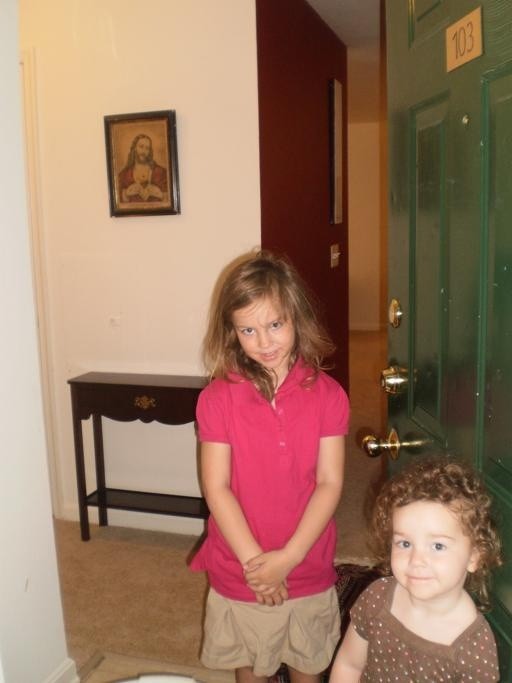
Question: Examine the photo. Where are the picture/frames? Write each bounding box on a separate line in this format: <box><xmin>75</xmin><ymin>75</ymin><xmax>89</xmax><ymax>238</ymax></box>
<box><xmin>103</xmin><ymin>109</ymin><xmax>182</xmax><ymax>219</ymax></box>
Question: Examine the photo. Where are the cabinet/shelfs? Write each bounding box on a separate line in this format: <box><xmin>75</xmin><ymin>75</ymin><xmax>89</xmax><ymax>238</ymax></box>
<box><xmin>67</xmin><ymin>370</ymin><xmax>215</xmax><ymax>543</ymax></box>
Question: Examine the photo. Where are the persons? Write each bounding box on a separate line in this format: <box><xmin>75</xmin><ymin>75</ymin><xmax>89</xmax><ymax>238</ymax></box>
<box><xmin>116</xmin><ymin>134</ymin><xmax>168</xmax><ymax>204</ymax></box>
<box><xmin>187</xmin><ymin>249</ymin><xmax>350</xmax><ymax>682</ymax></box>
<box><xmin>324</xmin><ymin>462</ymin><xmax>501</xmax><ymax>682</ymax></box>
